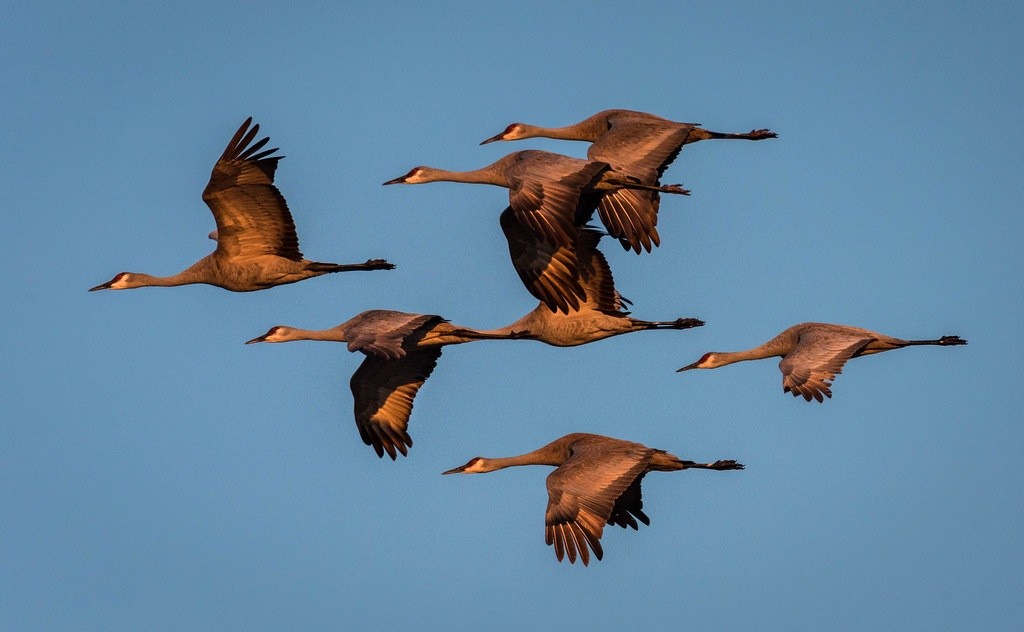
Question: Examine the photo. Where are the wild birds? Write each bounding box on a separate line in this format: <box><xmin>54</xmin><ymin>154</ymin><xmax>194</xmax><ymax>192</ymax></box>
<box><xmin>383</xmin><ymin>109</ymin><xmax>779</xmax><ymax>347</ymax></box>
<box><xmin>245</xmin><ymin>308</ymin><xmax>540</xmax><ymax>459</ymax></box>
<box><xmin>86</xmin><ymin>115</ymin><xmax>396</xmax><ymax>292</ymax></box>
<box><xmin>440</xmin><ymin>433</ymin><xmax>746</xmax><ymax>564</ymax></box>
<box><xmin>677</xmin><ymin>321</ymin><xmax>969</xmax><ymax>404</ymax></box>
